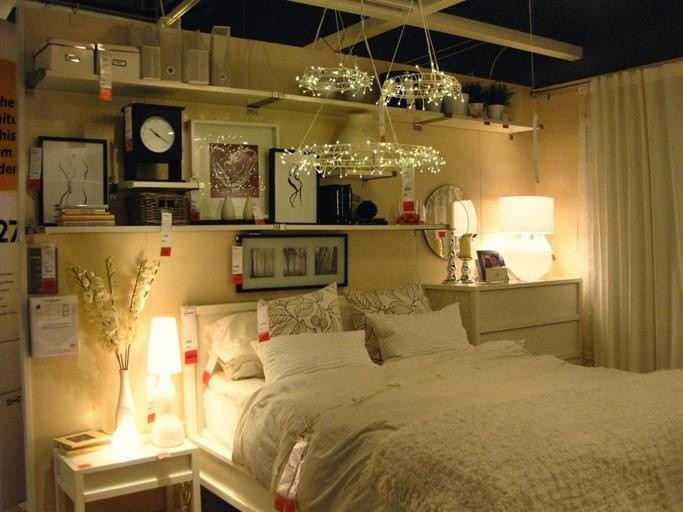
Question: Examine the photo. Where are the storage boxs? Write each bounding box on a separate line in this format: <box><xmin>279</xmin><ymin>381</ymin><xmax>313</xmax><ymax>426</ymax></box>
<box><xmin>95</xmin><ymin>43</ymin><xmax>140</xmax><ymax>80</ymax></box>
<box><xmin>33</xmin><ymin>38</ymin><xmax>95</xmax><ymax>76</ymax></box>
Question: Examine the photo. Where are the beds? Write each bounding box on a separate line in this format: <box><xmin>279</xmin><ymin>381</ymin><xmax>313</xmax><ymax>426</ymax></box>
<box><xmin>179</xmin><ymin>302</ymin><xmax>682</xmax><ymax>512</ymax></box>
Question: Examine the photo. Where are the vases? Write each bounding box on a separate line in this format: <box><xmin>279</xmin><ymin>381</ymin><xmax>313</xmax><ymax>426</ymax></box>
<box><xmin>242</xmin><ymin>196</ymin><xmax>254</xmax><ymax>220</ymax></box>
<box><xmin>422</xmin><ymin>95</ymin><xmax>444</xmax><ymax>113</ymax></box>
<box><xmin>442</xmin><ymin>92</ymin><xmax>470</xmax><ymax>117</ymax></box>
<box><xmin>221</xmin><ymin>195</ymin><xmax>235</xmax><ymax>220</ymax></box>
<box><xmin>114</xmin><ymin>368</ymin><xmax>137</xmax><ymax>435</ymax></box>
<box><xmin>338</xmin><ymin>86</ymin><xmax>366</xmax><ymax>104</ymax></box>
<box><xmin>374</xmin><ymin>70</ymin><xmax>423</xmax><ymax>111</ymax></box>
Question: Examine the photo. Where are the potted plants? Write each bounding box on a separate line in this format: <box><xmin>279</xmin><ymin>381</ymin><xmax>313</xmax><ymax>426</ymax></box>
<box><xmin>483</xmin><ymin>82</ymin><xmax>515</xmax><ymax>122</ymax></box>
<box><xmin>461</xmin><ymin>81</ymin><xmax>489</xmax><ymax>119</ymax></box>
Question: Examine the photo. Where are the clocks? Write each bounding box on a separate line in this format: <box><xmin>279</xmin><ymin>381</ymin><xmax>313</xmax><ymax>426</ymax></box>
<box><xmin>119</xmin><ymin>102</ymin><xmax>187</xmax><ymax>183</ymax></box>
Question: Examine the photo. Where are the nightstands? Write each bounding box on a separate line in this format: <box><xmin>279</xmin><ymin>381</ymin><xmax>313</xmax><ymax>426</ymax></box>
<box><xmin>421</xmin><ymin>278</ymin><xmax>583</xmax><ymax>366</ymax></box>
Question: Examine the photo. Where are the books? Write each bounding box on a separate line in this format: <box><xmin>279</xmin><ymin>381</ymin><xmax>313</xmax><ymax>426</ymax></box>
<box><xmin>53</xmin><ymin>202</ymin><xmax>115</xmax><ymax>225</ymax></box>
<box><xmin>53</xmin><ymin>430</ymin><xmax>111</xmax><ymax>458</ymax></box>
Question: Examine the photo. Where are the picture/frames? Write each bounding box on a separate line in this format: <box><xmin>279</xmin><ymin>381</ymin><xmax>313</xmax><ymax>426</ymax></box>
<box><xmin>268</xmin><ymin>149</ymin><xmax>319</xmax><ymax>225</ymax></box>
<box><xmin>38</xmin><ymin>136</ymin><xmax>108</xmax><ymax>227</ymax></box>
<box><xmin>475</xmin><ymin>249</ymin><xmax>510</xmax><ymax>282</ymax></box>
<box><xmin>235</xmin><ymin>233</ymin><xmax>348</xmax><ymax>294</ymax></box>
<box><xmin>189</xmin><ymin>118</ymin><xmax>280</xmax><ymax>220</ymax></box>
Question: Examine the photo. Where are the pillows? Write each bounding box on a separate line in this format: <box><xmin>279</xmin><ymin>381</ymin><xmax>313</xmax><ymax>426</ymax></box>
<box><xmin>203</xmin><ymin>311</ymin><xmax>264</xmax><ymax>381</ymax></box>
<box><xmin>256</xmin><ymin>281</ymin><xmax>343</xmax><ymax>340</ymax></box>
<box><xmin>342</xmin><ymin>278</ymin><xmax>428</xmax><ymax>363</ymax></box>
<box><xmin>248</xmin><ymin>329</ymin><xmax>372</xmax><ymax>384</ymax></box>
<box><xmin>365</xmin><ymin>302</ymin><xmax>469</xmax><ymax>366</ymax></box>
<box><xmin>337</xmin><ymin>295</ymin><xmax>353</xmax><ymax>331</ymax></box>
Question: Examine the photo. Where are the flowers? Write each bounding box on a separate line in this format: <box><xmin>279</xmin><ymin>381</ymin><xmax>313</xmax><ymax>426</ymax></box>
<box><xmin>66</xmin><ymin>257</ymin><xmax>160</xmax><ymax>370</ymax></box>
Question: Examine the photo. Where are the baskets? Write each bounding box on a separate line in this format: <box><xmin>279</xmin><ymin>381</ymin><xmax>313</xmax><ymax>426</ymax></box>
<box><xmin>125</xmin><ymin>193</ymin><xmax>189</xmax><ymax>224</ymax></box>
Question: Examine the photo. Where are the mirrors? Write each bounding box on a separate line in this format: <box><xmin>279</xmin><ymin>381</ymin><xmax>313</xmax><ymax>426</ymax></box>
<box><xmin>423</xmin><ymin>185</ymin><xmax>476</xmax><ymax>260</ymax></box>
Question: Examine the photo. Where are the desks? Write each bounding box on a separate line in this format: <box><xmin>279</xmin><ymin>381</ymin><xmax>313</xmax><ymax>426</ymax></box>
<box><xmin>51</xmin><ymin>431</ymin><xmax>202</xmax><ymax>512</ymax></box>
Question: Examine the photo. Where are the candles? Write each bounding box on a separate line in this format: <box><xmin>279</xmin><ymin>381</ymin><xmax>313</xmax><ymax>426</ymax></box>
<box><xmin>458</xmin><ymin>237</ymin><xmax>471</xmax><ymax>257</ymax></box>
<box><xmin>449</xmin><ymin>203</ymin><xmax>455</xmax><ymax>229</ymax></box>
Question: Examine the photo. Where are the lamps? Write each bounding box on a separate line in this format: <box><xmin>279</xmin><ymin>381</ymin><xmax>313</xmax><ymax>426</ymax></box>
<box><xmin>452</xmin><ymin>199</ymin><xmax>477</xmax><ymax>235</ymax></box>
<box><xmin>497</xmin><ymin>195</ymin><xmax>554</xmax><ymax>282</ymax></box>
<box><xmin>295</xmin><ymin>0</ymin><xmax>374</xmax><ymax>98</ymax></box>
<box><xmin>145</xmin><ymin>316</ymin><xmax>183</xmax><ymax>447</ymax></box>
<box><xmin>278</xmin><ymin>1</ymin><xmax>447</xmax><ymax>180</ymax></box>
<box><xmin>374</xmin><ymin>0</ymin><xmax>465</xmax><ymax>111</ymax></box>
